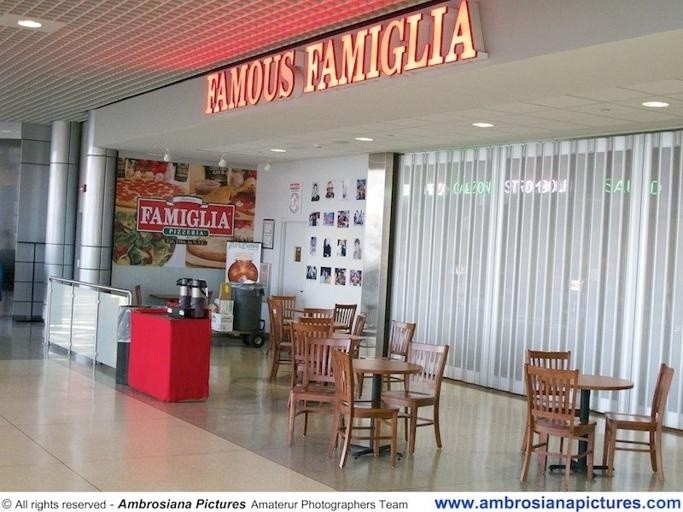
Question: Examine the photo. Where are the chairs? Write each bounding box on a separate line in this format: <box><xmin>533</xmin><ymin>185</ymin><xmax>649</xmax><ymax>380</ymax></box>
<box><xmin>265</xmin><ymin>296</ymin><xmax>448</xmax><ymax>470</ymax></box>
<box><xmin>520</xmin><ymin>352</ymin><xmax>674</xmax><ymax>482</ymax></box>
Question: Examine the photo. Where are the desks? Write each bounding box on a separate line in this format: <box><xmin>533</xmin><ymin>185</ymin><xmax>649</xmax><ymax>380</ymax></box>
<box><xmin>149</xmin><ymin>294</ymin><xmax>179</xmax><ymax>300</ymax></box>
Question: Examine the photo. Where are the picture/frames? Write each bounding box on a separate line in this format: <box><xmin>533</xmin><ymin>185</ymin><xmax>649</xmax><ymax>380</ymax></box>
<box><xmin>261</xmin><ymin>219</ymin><xmax>275</xmax><ymax>249</ymax></box>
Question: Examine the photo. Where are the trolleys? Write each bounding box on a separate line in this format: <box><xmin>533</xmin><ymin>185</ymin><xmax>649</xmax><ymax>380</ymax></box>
<box><xmin>210</xmin><ymin>319</ymin><xmax>266</xmax><ymax>348</ymax></box>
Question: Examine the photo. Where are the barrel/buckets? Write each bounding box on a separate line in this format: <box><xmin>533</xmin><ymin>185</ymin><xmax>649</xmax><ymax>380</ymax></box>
<box><xmin>231</xmin><ymin>284</ymin><xmax>265</xmax><ymax>332</ymax></box>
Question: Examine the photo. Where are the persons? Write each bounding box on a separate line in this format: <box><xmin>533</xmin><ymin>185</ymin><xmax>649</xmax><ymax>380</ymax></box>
<box><xmin>325</xmin><ymin>181</ymin><xmax>335</xmax><ymax>198</ymax></box>
<box><xmin>351</xmin><ymin>239</ymin><xmax>362</xmax><ymax>261</ymax></box>
<box><xmin>312</xmin><ymin>183</ymin><xmax>320</xmax><ymax>201</ymax></box>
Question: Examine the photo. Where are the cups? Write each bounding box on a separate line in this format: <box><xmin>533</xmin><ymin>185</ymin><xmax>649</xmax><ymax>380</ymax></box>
<box><xmin>192</xmin><ymin>180</ymin><xmax>221</xmax><ymax>195</ymax></box>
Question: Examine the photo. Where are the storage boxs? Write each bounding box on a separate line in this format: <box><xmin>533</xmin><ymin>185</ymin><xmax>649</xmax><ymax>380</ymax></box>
<box><xmin>211</xmin><ymin>298</ymin><xmax>234</xmax><ymax>332</ymax></box>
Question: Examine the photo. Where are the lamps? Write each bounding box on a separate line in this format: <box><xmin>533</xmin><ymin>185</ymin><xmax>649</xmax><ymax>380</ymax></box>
<box><xmin>164</xmin><ymin>151</ymin><xmax>273</xmax><ymax>172</ymax></box>
